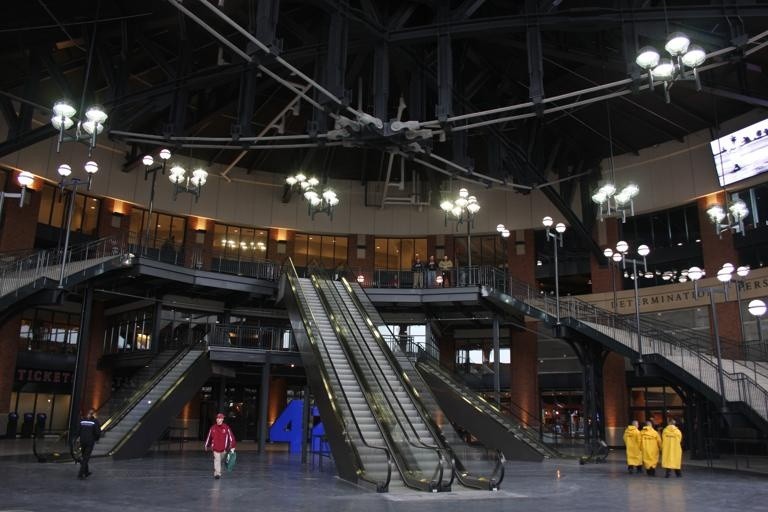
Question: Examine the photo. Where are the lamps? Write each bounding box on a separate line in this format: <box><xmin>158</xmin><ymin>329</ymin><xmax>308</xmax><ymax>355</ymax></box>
<box><xmin>706</xmin><ymin>199</ymin><xmax>750</xmax><ymax>233</ymax></box>
<box><xmin>542</xmin><ymin>216</ymin><xmax>554</xmax><ymax>228</ymax></box>
<box><xmin>592</xmin><ymin>183</ymin><xmax>641</xmax><ymax>206</ymax></box>
<box><xmin>285</xmin><ymin>173</ymin><xmax>340</xmax><ymax>207</ymax></box>
<box><xmin>440</xmin><ymin>185</ymin><xmax>483</xmax><ymax>222</ymax></box>
<box><xmin>17</xmin><ymin>103</ymin><xmax>209</xmax><ymax>188</ymax></box>
<box><xmin>635</xmin><ymin>32</ymin><xmax>707</xmax><ymax>82</ymax></box>
<box><xmin>555</xmin><ymin>222</ymin><xmax>566</xmax><ymax>233</ymax></box>
<box><xmin>496</xmin><ymin>223</ymin><xmax>511</xmax><ymax>238</ymax></box>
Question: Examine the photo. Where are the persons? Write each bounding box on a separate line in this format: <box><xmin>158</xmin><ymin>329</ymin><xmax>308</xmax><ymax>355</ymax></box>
<box><xmin>425</xmin><ymin>254</ymin><xmax>439</xmax><ymax>289</ymax></box>
<box><xmin>640</xmin><ymin>420</ymin><xmax>663</xmax><ymax>476</ymax></box>
<box><xmin>412</xmin><ymin>255</ymin><xmax>426</xmax><ymax>289</ymax></box>
<box><xmin>622</xmin><ymin>419</ymin><xmax>644</xmax><ymax>475</ymax></box>
<box><xmin>438</xmin><ymin>254</ymin><xmax>453</xmax><ymax>288</ymax></box>
<box><xmin>73</xmin><ymin>407</ymin><xmax>102</xmax><ymax>480</ymax></box>
<box><xmin>205</xmin><ymin>413</ymin><xmax>237</xmax><ymax>480</ymax></box>
<box><xmin>660</xmin><ymin>417</ymin><xmax>683</xmax><ymax>479</ymax></box>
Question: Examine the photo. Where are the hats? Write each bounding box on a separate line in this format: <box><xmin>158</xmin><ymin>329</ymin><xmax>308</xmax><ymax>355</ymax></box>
<box><xmin>216</xmin><ymin>414</ymin><xmax>224</xmax><ymax>418</ymax></box>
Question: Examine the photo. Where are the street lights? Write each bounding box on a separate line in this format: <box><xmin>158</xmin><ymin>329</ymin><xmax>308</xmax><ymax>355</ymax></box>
<box><xmin>459</xmin><ymin>187</ymin><xmax>478</xmax><ymax>285</ymax></box>
<box><xmin>543</xmin><ymin>215</ymin><xmax>566</xmax><ymax>326</ymax></box>
<box><xmin>143</xmin><ymin>148</ymin><xmax>172</xmax><ymax>256</ymax></box>
<box><xmin>496</xmin><ymin>223</ymin><xmax>511</xmax><ymax>294</ymax></box>
<box><xmin>686</xmin><ymin>266</ymin><xmax>732</xmax><ymax>414</ymax></box>
<box><xmin>722</xmin><ymin>262</ymin><xmax>750</xmax><ymax>361</ymax></box>
<box><xmin>56</xmin><ymin>160</ymin><xmax>99</xmax><ymax>289</ymax></box>
<box><xmin>603</xmin><ymin>246</ymin><xmax>622</xmax><ymax>315</ymax></box>
<box><xmin>616</xmin><ymin>240</ymin><xmax>651</xmax><ymax>360</ymax></box>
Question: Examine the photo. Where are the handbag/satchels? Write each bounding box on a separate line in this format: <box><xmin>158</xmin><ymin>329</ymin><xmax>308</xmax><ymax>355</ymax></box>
<box><xmin>226</xmin><ymin>451</ymin><xmax>236</xmax><ymax>472</ymax></box>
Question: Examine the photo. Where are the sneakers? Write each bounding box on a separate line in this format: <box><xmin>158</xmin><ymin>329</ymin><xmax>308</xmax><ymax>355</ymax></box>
<box><xmin>215</xmin><ymin>475</ymin><xmax>220</xmax><ymax>479</ymax></box>
<box><xmin>628</xmin><ymin>471</ymin><xmax>681</xmax><ymax>478</ymax></box>
